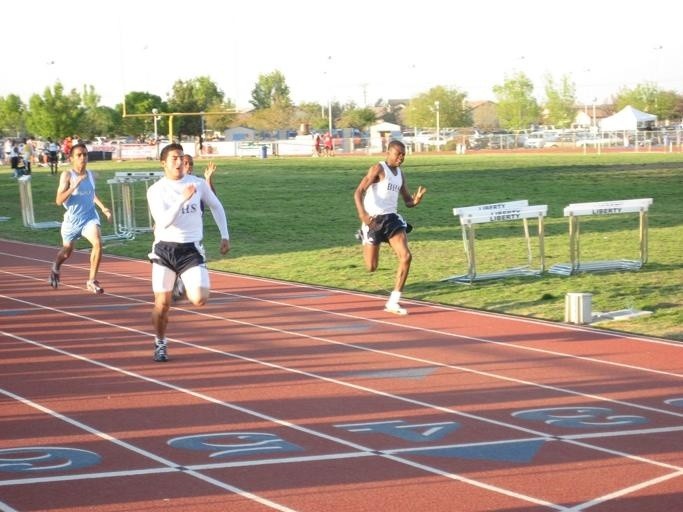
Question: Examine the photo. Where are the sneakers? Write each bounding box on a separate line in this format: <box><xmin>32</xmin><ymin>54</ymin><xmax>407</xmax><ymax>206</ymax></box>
<box><xmin>383</xmin><ymin>301</ymin><xmax>409</xmax><ymax>316</ymax></box>
<box><xmin>87</xmin><ymin>280</ymin><xmax>104</xmax><ymax>296</ymax></box>
<box><xmin>355</xmin><ymin>221</ymin><xmax>370</xmax><ymax>241</ymax></box>
<box><xmin>49</xmin><ymin>264</ymin><xmax>61</xmax><ymax>289</ymax></box>
<box><xmin>153</xmin><ymin>337</ymin><xmax>168</xmax><ymax>362</ymax></box>
<box><xmin>171</xmin><ymin>276</ymin><xmax>186</xmax><ymax>303</ymax></box>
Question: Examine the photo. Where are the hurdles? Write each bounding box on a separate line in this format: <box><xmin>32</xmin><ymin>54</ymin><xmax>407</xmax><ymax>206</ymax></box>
<box><xmin>551</xmin><ymin>198</ymin><xmax>655</xmax><ymax>276</ymax></box>
<box><xmin>452</xmin><ymin>200</ymin><xmax>549</xmax><ymax>284</ymax></box>
<box><xmin>107</xmin><ymin>169</ymin><xmax>169</xmax><ymax>238</ymax></box>
<box><xmin>17</xmin><ymin>174</ymin><xmax>61</xmax><ymax>230</ymax></box>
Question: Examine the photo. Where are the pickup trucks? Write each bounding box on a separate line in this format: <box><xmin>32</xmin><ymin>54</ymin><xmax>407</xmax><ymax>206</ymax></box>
<box><xmin>103</xmin><ymin>136</ymin><xmax>135</xmax><ymax>145</ymax></box>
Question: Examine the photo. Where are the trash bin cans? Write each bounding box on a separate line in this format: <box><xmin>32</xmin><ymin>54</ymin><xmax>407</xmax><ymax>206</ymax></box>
<box><xmin>260</xmin><ymin>147</ymin><xmax>266</xmax><ymax>158</ymax></box>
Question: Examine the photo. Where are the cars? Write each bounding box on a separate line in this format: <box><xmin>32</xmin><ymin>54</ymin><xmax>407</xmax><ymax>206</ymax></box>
<box><xmin>403</xmin><ymin>124</ymin><xmax>622</xmax><ymax>149</ymax></box>
<box><xmin>333</xmin><ymin>129</ymin><xmax>362</xmax><ymax>148</ymax></box>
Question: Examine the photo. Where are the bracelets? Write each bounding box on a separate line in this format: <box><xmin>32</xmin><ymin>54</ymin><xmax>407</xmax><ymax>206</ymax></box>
<box><xmin>101</xmin><ymin>207</ymin><xmax>110</xmax><ymax>216</ymax></box>
<box><xmin>70</xmin><ymin>182</ymin><xmax>78</xmax><ymax>189</ymax></box>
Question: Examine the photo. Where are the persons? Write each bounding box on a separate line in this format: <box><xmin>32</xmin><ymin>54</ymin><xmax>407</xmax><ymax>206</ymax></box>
<box><xmin>138</xmin><ymin>132</ymin><xmax>231</xmax><ymax>362</ymax></box>
<box><xmin>0</xmin><ymin>134</ymin><xmax>84</xmax><ymax>178</ymax></box>
<box><xmin>313</xmin><ymin>131</ymin><xmax>335</xmax><ymax>156</ymax></box>
<box><xmin>47</xmin><ymin>144</ymin><xmax>113</xmax><ymax>295</ymax></box>
<box><xmin>353</xmin><ymin>139</ymin><xmax>428</xmax><ymax>316</ymax></box>
